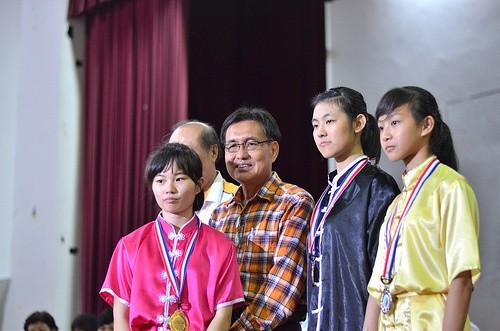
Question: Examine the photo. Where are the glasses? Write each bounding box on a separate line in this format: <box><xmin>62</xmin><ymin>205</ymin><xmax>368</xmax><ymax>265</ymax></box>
<box><xmin>225</xmin><ymin>139</ymin><xmax>274</xmax><ymax>153</ymax></box>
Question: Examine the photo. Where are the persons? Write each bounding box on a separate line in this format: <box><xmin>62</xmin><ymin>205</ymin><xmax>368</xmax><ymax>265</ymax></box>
<box><xmin>71</xmin><ymin>307</ymin><xmax>114</xmax><ymax>331</ymax></box>
<box><xmin>99</xmin><ymin>142</ymin><xmax>245</xmax><ymax>331</ymax></box>
<box><xmin>306</xmin><ymin>86</ymin><xmax>402</xmax><ymax>331</ymax></box>
<box><xmin>361</xmin><ymin>86</ymin><xmax>481</xmax><ymax>331</ymax></box>
<box><xmin>168</xmin><ymin>120</ymin><xmax>239</xmax><ymax>226</ymax></box>
<box><xmin>208</xmin><ymin>108</ymin><xmax>316</xmax><ymax>331</ymax></box>
<box><xmin>24</xmin><ymin>311</ymin><xmax>59</xmax><ymax>331</ymax></box>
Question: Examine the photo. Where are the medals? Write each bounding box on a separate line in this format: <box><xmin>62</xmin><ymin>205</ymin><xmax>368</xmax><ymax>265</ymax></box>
<box><xmin>378</xmin><ymin>288</ymin><xmax>393</xmax><ymax>313</ymax></box>
<box><xmin>308</xmin><ymin>260</ymin><xmax>318</xmax><ymax>283</ymax></box>
<box><xmin>169</xmin><ymin>308</ymin><xmax>189</xmax><ymax>331</ymax></box>
